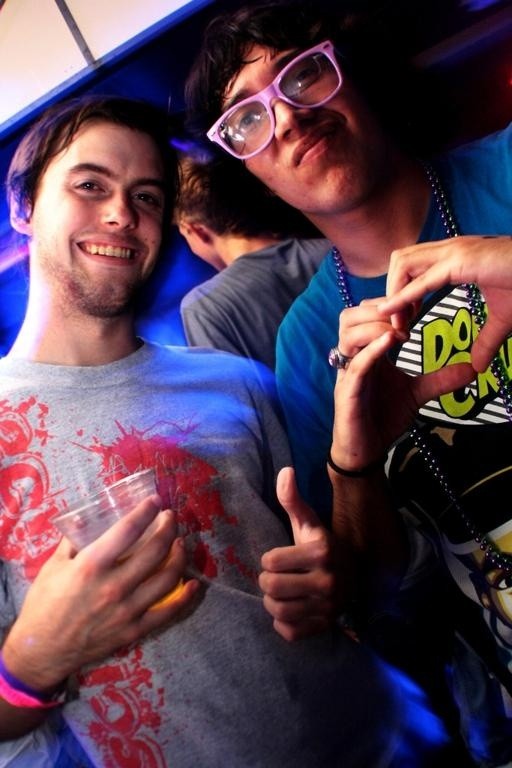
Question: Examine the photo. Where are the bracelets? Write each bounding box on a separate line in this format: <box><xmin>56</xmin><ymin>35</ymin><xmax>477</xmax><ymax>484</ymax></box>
<box><xmin>0</xmin><ymin>647</ymin><xmax>68</xmax><ymax>713</ymax></box>
<box><xmin>326</xmin><ymin>447</ymin><xmax>371</xmax><ymax>483</ymax></box>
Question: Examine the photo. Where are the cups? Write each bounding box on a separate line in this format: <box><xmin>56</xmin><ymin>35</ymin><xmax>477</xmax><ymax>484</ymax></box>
<box><xmin>49</xmin><ymin>468</ymin><xmax>185</xmax><ymax>614</ymax></box>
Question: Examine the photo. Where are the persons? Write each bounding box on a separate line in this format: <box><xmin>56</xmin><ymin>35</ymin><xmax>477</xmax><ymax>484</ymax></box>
<box><xmin>169</xmin><ymin>3</ymin><xmax>511</xmax><ymax>710</ymax></box>
<box><xmin>167</xmin><ymin>147</ymin><xmax>511</xmax><ymax>764</ymax></box>
<box><xmin>0</xmin><ymin>95</ymin><xmax>411</xmax><ymax>765</ymax></box>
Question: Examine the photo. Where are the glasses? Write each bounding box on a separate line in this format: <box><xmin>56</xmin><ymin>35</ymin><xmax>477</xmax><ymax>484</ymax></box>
<box><xmin>204</xmin><ymin>40</ymin><xmax>345</xmax><ymax>160</ymax></box>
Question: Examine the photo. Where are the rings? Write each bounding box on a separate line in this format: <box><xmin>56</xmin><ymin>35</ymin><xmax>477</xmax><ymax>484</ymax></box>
<box><xmin>324</xmin><ymin>349</ymin><xmax>353</xmax><ymax>370</ymax></box>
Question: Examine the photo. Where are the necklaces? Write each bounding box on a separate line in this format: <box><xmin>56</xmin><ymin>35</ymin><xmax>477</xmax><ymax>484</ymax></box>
<box><xmin>328</xmin><ymin>163</ymin><xmax>510</xmax><ymax>577</ymax></box>
